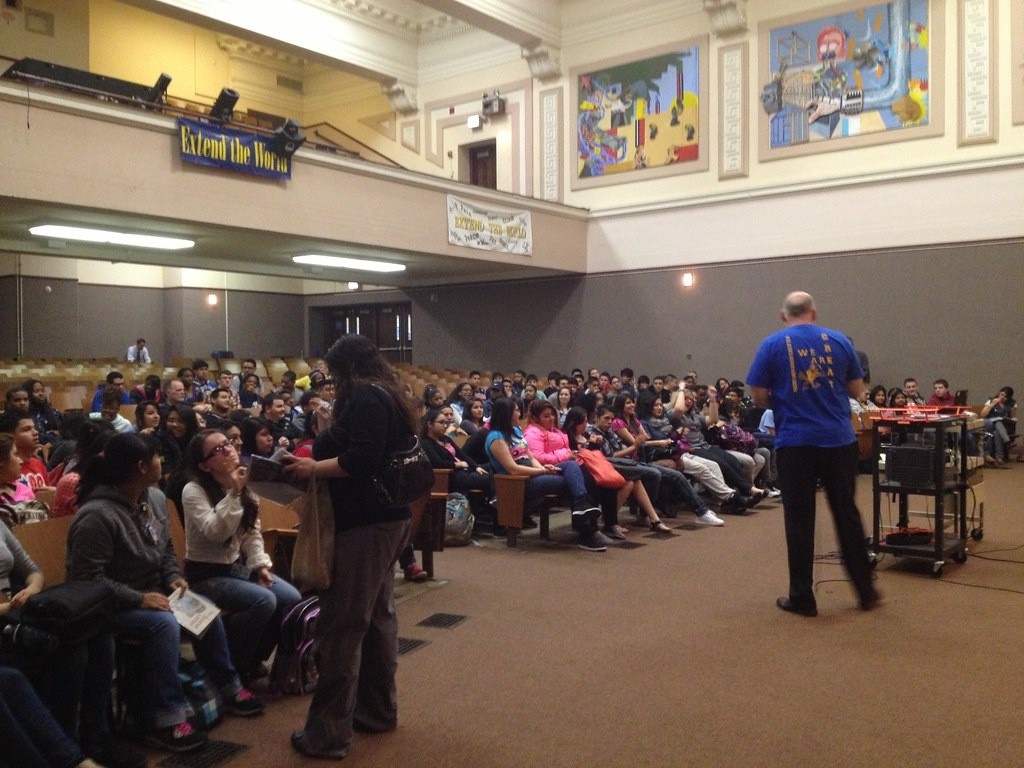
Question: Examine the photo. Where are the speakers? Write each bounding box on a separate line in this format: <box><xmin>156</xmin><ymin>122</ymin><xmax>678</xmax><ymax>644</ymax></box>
<box><xmin>885</xmin><ymin>442</ymin><xmax>937</xmax><ymax>487</ymax></box>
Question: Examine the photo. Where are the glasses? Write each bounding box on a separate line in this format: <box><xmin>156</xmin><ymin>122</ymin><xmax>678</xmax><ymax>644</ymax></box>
<box><xmin>431</xmin><ymin>419</ymin><xmax>449</xmax><ymax>426</ymax></box>
<box><xmin>201</xmin><ymin>438</ymin><xmax>235</xmax><ymax>464</ymax></box>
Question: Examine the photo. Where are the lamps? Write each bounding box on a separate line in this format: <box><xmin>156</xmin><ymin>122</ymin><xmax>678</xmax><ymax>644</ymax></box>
<box><xmin>480</xmin><ymin>89</ymin><xmax>505</xmax><ymax>115</ymax></box>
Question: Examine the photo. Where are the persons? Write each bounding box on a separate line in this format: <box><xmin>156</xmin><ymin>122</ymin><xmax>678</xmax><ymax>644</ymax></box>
<box><xmin>280</xmin><ymin>334</ymin><xmax>413</xmax><ymax>757</ymax></box>
<box><xmin>842</xmin><ymin>336</ymin><xmax>1017</xmax><ymax>468</ymax></box>
<box><xmin>128</xmin><ymin>338</ymin><xmax>152</xmax><ymax>364</ymax></box>
<box><xmin>746</xmin><ymin>292</ymin><xmax>887</xmax><ymax>617</ymax></box>
<box><xmin>0</xmin><ymin>359</ymin><xmax>776</xmax><ymax>768</ymax></box>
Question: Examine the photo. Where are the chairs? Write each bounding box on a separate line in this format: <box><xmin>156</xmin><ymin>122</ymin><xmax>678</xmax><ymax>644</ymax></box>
<box><xmin>0</xmin><ymin>347</ymin><xmax>534</xmax><ymax>589</ymax></box>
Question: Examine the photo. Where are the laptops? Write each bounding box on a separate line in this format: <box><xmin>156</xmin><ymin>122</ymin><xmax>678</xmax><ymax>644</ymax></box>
<box><xmin>954</xmin><ymin>389</ymin><xmax>968</xmax><ymax>406</ymax></box>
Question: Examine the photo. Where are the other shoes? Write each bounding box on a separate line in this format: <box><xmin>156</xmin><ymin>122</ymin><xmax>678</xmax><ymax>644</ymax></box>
<box><xmin>594</xmin><ymin>530</ymin><xmax>614</xmax><ymax>544</ymax></box>
<box><xmin>90</xmin><ymin>743</ymin><xmax>148</xmax><ymax>768</ymax></box>
<box><xmin>403</xmin><ymin>564</ymin><xmax>428</xmax><ymax>581</ymax></box>
<box><xmin>492</xmin><ymin>525</ymin><xmax>523</xmax><ymax>539</ymax></box>
<box><xmin>767</xmin><ymin>486</ymin><xmax>781</xmax><ymax>497</ymax></box>
<box><xmin>222</xmin><ymin>689</ymin><xmax>265</xmax><ymax>716</ymax></box>
<box><xmin>577</xmin><ymin>536</ymin><xmax>606</xmax><ymax>551</ymax></box>
<box><xmin>572</xmin><ymin>494</ymin><xmax>603</xmax><ymax>523</ymax></box>
<box><xmin>489</xmin><ymin>496</ymin><xmax>498</xmax><ymax>509</ymax></box>
<box><xmin>637</xmin><ymin>514</ymin><xmax>651</xmax><ymax>526</ymax></box>
<box><xmin>776</xmin><ymin>597</ymin><xmax>818</xmax><ymax>617</ymax></box>
<box><xmin>523</xmin><ymin>513</ymin><xmax>537</xmax><ymax>527</ymax></box>
<box><xmin>695</xmin><ymin>509</ymin><xmax>725</xmax><ymax>526</ymax></box>
<box><xmin>604</xmin><ymin>525</ymin><xmax>626</xmax><ymax>539</ymax></box>
<box><xmin>145</xmin><ymin>722</ymin><xmax>204</xmax><ymax>752</ymax></box>
<box><xmin>720</xmin><ymin>490</ymin><xmax>762</xmax><ymax>514</ymax></box>
<box><xmin>237</xmin><ymin>657</ymin><xmax>268</xmax><ymax>684</ymax></box>
<box><xmin>750</xmin><ymin>485</ymin><xmax>768</xmax><ymax>499</ymax></box>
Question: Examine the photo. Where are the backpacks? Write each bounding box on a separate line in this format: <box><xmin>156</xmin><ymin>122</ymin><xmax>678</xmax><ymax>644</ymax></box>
<box><xmin>441</xmin><ymin>491</ymin><xmax>475</xmax><ymax>547</ymax></box>
<box><xmin>277</xmin><ymin>592</ymin><xmax>322</xmax><ymax>695</ymax></box>
<box><xmin>721</xmin><ymin>423</ymin><xmax>759</xmax><ymax>453</ymax></box>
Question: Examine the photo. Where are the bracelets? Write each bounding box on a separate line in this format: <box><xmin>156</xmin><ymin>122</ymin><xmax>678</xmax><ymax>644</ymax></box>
<box><xmin>678</xmin><ymin>389</ymin><xmax>685</xmax><ymax>392</ymax></box>
<box><xmin>460</xmin><ymin>461</ymin><xmax>464</xmax><ymax>468</ymax></box>
<box><xmin>541</xmin><ymin>466</ymin><xmax>545</xmax><ymax>475</ymax></box>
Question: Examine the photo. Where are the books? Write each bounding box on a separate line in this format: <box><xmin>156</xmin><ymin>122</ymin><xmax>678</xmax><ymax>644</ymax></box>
<box><xmin>166</xmin><ymin>586</ymin><xmax>222</xmax><ymax>642</ymax></box>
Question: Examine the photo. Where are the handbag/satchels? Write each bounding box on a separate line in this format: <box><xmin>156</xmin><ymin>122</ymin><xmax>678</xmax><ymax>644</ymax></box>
<box><xmin>576</xmin><ymin>446</ymin><xmax>626</xmax><ymax>489</ymax></box>
<box><xmin>18</xmin><ymin>577</ymin><xmax>121</xmax><ymax>651</ymax></box>
<box><xmin>645</xmin><ymin>429</ymin><xmax>681</xmax><ymax>463</ymax></box>
<box><xmin>289</xmin><ymin>462</ymin><xmax>337</xmax><ymax>593</ymax></box>
<box><xmin>122</xmin><ymin>651</ymin><xmax>226</xmax><ymax>732</ymax></box>
<box><xmin>353</xmin><ymin>382</ymin><xmax>437</xmax><ymax>510</ymax></box>
<box><xmin>661</xmin><ymin>429</ymin><xmax>690</xmax><ymax>461</ymax></box>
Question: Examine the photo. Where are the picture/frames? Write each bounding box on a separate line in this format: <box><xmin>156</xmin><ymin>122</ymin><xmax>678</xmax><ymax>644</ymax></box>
<box><xmin>757</xmin><ymin>0</ymin><xmax>946</xmax><ymax>163</ymax></box>
<box><xmin>570</xmin><ymin>33</ymin><xmax>710</xmax><ymax>191</ymax></box>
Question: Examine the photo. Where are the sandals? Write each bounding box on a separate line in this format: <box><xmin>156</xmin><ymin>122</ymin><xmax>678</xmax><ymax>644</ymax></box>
<box><xmin>649</xmin><ymin>521</ymin><xmax>672</xmax><ymax>532</ymax></box>
<box><xmin>618</xmin><ymin>523</ymin><xmax>630</xmax><ymax>532</ymax></box>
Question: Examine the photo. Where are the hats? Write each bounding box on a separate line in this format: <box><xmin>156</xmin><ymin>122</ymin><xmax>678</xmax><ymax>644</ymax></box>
<box><xmin>491</xmin><ymin>382</ymin><xmax>503</xmax><ymax>391</ymax></box>
<box><xmin>308</xmin><ymin>368</ymin><xmax>319</xmax><ymax>380</ymax></box>
